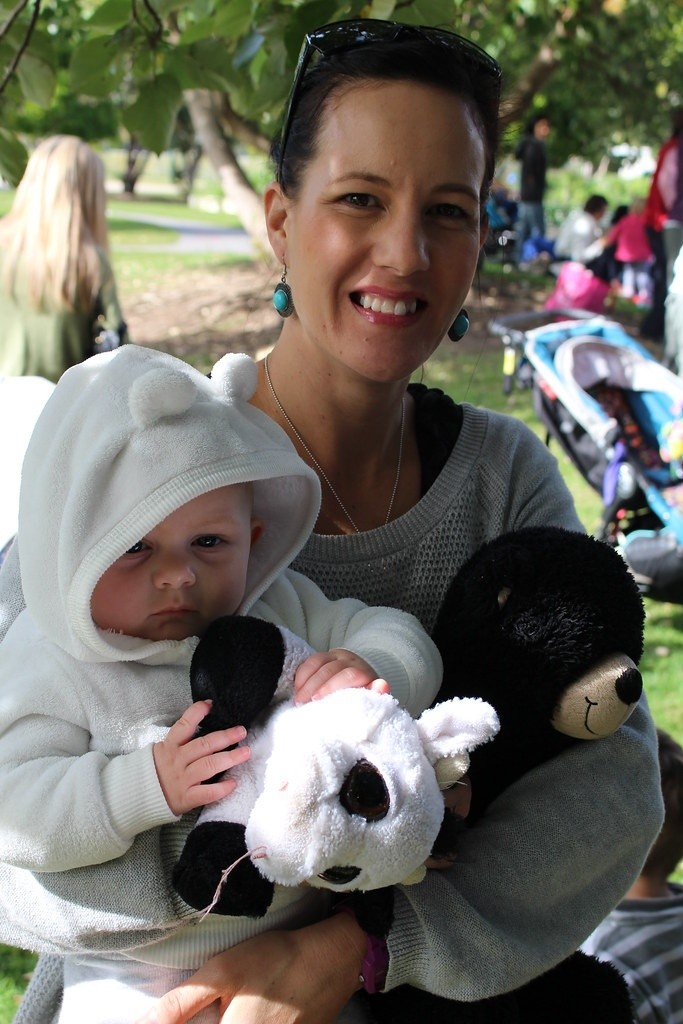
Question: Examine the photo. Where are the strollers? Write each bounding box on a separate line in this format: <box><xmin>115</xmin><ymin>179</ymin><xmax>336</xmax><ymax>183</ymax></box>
<box><xmin>492</xmin><ymin>307</ymin><xmax>683</xmax><ymax>596</ymax></box>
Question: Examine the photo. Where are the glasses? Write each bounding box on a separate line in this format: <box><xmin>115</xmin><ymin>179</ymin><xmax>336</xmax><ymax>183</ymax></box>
<box><xmin>276</xmin><ymin>16</ymin><xmax>503</xmax><ymax>184</ymax></box>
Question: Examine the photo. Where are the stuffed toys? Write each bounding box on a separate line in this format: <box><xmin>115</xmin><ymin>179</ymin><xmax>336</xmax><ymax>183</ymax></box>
<box><xmin>432</xmin><ymin>524</ymin><xmax>646</xmax><ymax>860</ymax></box>
<box><xmin>174</xmin><ymin>614</ymin><xmax>500</xmax><ymax>939</ymax></box>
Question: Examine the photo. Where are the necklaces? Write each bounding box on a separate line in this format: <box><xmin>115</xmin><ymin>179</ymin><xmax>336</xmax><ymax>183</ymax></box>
<box><xmin>265</xmin><ymin>353</ymin><xmax>406</xmax><ymax>589</ymax></box>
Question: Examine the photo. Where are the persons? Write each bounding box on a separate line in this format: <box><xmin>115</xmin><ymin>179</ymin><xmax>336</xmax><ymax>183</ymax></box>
<box><xmin>553</xmin><ymin>194</ymin><xmax>655</xmax><ymax>302</ymax></box>
<box><xmin>574</xmin><ymin>726</ymin><xmax>682</xmax><ymax>1022</ymax></box>
<box><xmin>4</xmin><ymin>16</ymin><xmax>665</xmax><ymax>1023</ymax></box>
<box><xmin>1</xmin><ymin>136</ymin><xmax>129</xmax><ymax>387</ymax></box>
<box><xmin>513</xmin><ymin>117</ymin><xmax>550</xmax><ymax>264</ymax></box>
<box><xmin>0</xmin><ymin>343</ymin><xmax>442</xmax><ymax>1024</ymax></box>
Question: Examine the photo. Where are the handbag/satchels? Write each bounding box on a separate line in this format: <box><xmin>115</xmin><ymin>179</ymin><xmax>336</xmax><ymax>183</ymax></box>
<box><xmin>544</xmin><ymin>260</ymin><xmax>617</xmax><ymax>324</ymax></box>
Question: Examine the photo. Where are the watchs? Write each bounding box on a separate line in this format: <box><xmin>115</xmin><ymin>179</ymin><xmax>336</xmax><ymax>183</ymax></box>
<box><xmin>360</xmin><ymin>933</ymin><xmax>388</xmax><ymax>994</ymax></box>
